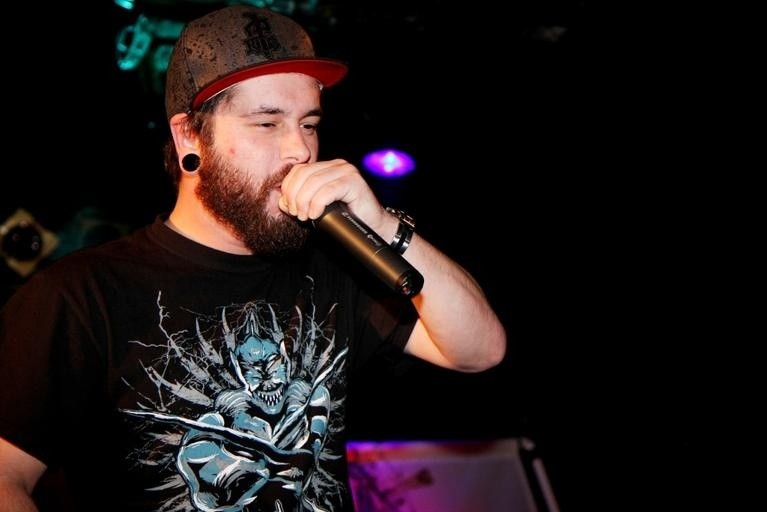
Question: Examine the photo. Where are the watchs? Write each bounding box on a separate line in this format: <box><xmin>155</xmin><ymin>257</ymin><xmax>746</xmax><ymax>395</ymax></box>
<box><xmin>388</xmin><ymin>205</ymin><xmax>417</xmax><ymax>255</ymax></box>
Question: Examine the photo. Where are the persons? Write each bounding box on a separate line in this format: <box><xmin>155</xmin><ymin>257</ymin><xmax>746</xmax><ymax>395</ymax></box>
<box><xmin>0</xmin><ymin>3</ymin><xmax>511</xmax><ymax>511</ymax></box>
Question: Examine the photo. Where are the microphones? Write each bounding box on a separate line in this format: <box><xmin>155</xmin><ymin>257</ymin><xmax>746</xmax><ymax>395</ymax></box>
<box><xmin>310</xmin><ymin>201</ymin><xmax>425</xmax><ymax>301</ymax></box>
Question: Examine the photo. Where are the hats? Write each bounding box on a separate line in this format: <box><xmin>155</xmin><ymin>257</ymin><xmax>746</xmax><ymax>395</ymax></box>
<box><xmin>165</xmin><ymin>4</ymin><xmax>347</xmax><ymax>122</ymax></box>
